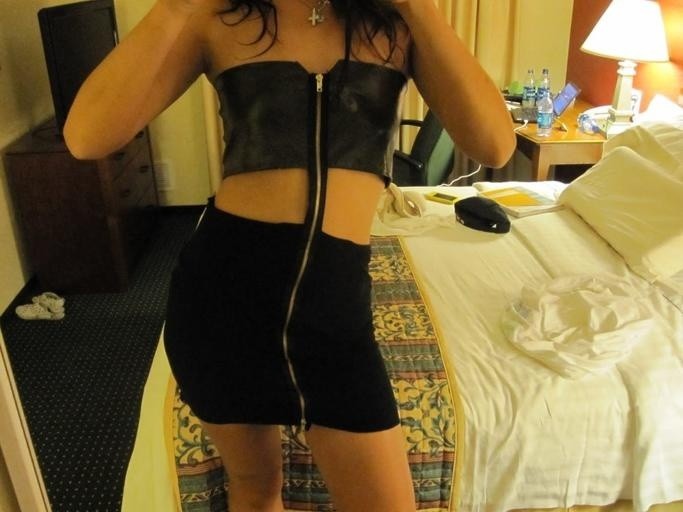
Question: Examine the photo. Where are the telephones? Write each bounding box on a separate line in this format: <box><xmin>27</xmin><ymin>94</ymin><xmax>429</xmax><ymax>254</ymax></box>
<box><xmin>577</xmin><ymin>104</ymin><xmax>613</xmax><ymax>128</ymax></box>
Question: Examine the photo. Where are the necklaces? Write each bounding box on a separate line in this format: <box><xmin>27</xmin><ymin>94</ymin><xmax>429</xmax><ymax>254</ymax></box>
<box><xmin>301</xmin><ymin>0</ymin><xmax>329</xmax><ymax>28</ymax></box>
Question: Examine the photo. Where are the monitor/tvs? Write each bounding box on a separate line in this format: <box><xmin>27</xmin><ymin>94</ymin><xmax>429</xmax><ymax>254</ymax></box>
<box><xmin>37</xmin><ymin>0</ymin><xmax>120</xmax><ymax>137</ymax></box>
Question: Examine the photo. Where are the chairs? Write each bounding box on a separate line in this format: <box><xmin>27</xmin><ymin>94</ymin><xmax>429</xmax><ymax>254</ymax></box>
<box><xmin>392</xmin><ymin>108</ymin><xmax>454</xmax><ymax>187</ymax></box>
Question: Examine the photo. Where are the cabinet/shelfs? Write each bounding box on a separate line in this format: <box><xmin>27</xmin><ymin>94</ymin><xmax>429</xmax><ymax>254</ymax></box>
<box><xmin>0</xmin><ymin>116</ymin><xmax>160</xmax><ymax>295</ymax></box>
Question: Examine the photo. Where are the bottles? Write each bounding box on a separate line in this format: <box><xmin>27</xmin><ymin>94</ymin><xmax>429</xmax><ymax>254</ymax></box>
<box><xmin>576</xmin><ymin>113</ymin><xmax>599</xmax><ymax>135</ymax></box>
<box><xmin>537</xmin><ymin>90</ymin><xmax>553</xmax><ymax>136</ymax></box>
<box><xmin>522</xmin><ymin>69</ymin><xmax>537</xmax><ymax>107</ymax></box>
<box><xmin>537</xmin><ymin>69</ymin><xmax>551</xmax><ymax>100</ymax></box>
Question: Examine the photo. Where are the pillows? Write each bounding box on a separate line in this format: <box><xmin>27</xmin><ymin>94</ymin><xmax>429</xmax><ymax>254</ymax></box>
<box><xmin>602</xmin><ymin>125</ymin><xmax>683</xmax><ymax>183</ymax></box>
<box><xmin>557</xmin><ymin>145</ymin><xmax>683</xmax><ymax>285</ymax></box>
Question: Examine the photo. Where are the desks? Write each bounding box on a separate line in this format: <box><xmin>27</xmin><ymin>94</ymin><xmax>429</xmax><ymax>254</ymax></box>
<box><xmin>486</xmin><ymin>94</ymin><xmax>607</xmax><ymax>181</ymax></box>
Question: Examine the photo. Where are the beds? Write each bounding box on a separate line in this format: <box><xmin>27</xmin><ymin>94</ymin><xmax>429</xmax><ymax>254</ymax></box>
<box><xmin>121</xmin><ymin>125</ymin><xmax>683</xmax><ymax>512</ymax></box>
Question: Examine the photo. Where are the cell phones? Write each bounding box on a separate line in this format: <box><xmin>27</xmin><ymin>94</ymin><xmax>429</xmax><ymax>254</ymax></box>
<box><xmin>425</xmin><ymin>190</ymin><xmax>459</xmax><ymax>205</ymax></box>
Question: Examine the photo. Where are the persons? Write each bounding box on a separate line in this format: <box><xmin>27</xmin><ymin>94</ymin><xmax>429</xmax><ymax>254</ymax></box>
<box><xmin>62</xmin><ymin>0</ymin><xmax>517</xmax><ymax>511</ymax></box>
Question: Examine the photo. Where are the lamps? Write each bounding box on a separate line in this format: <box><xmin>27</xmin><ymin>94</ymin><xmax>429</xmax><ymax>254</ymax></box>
<box><xmin>578</xmin><ymin>0</ymin><xmax>670</xmax><ymax>140</ymax></box>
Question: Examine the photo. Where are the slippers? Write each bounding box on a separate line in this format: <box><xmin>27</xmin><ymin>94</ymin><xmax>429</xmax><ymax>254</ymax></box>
<box><xmin>32</xmin><ymin>292</ymin><xmax>65</xmax><ymax>312</ymax></box>
<box><xmin>15</xmin><ymin>304</ymin><xmax>66</xmax><ymax>321</ymax></box>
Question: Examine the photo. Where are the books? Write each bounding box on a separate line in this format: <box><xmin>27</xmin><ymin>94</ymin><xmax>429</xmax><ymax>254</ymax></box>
<box><xmin>478</xmin><ymin>186</ymin><xmax>565</xmax><ymax>218</ymax></box>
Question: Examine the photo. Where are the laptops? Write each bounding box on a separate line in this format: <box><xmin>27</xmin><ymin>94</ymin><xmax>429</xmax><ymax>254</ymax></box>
<box><xmin>512</xmin><ymin>80</ymin><xmax>582</xmax><ymax>124</ymax></box>
